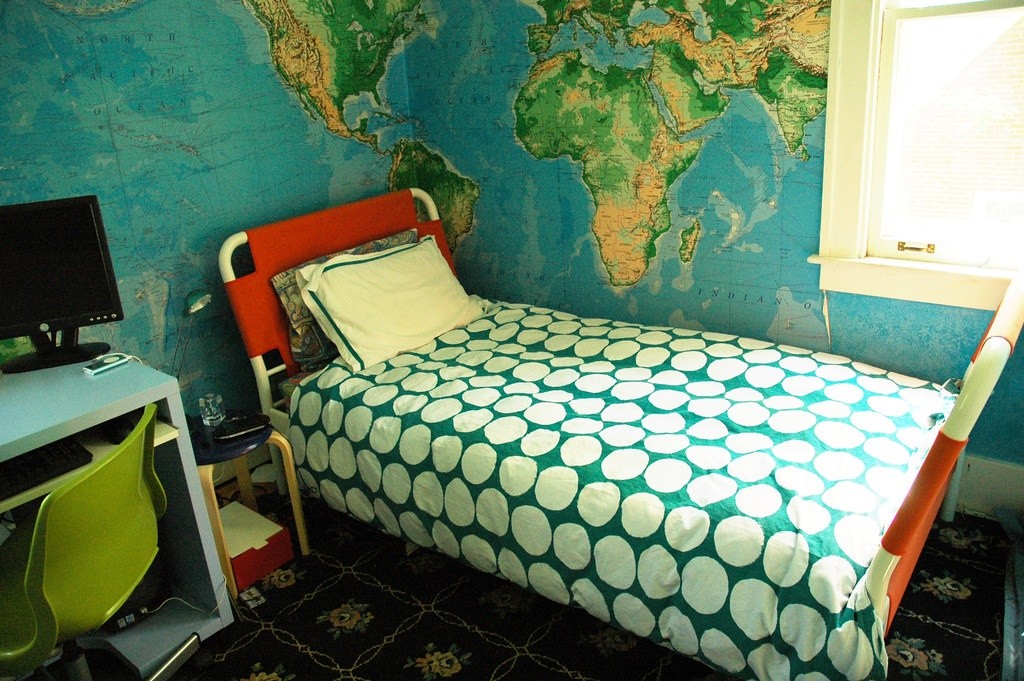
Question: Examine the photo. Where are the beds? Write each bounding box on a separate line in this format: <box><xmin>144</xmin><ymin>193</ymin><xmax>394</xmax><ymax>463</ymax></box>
<box><xmin>220</xmin><ymin>189</ymin><xmax>1024</xmax><ymax>681</ymax></box>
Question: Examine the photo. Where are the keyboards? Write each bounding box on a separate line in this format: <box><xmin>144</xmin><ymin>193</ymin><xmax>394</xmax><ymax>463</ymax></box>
<box><xmin>0</xmin><ymin>435</ymin><xmax>94</xmax><ymax>501</ymax></box>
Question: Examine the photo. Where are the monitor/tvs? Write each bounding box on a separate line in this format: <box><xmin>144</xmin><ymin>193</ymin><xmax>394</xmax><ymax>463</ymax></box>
<box><xmin>0</xmin><ymin>196</ymin><xmax>124</xmax><ymax>374</ymax></box>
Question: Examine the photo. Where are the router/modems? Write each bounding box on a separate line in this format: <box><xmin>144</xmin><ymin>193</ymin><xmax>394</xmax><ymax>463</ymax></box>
<box><xmin>101</xmin><ymin>569</ymin><xmax>173</xmax><ymax>635</ymax></box>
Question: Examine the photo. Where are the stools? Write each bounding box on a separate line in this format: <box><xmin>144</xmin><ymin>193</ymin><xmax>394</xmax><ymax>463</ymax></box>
<box><xmin>190</xmin><ymin>413</ymin><xmax>312</xmax><ymax>597</ymax></box>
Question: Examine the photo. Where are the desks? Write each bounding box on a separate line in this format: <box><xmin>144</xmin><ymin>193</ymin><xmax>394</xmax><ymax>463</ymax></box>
<box><xmin>0</xmin><ymin>355</ymin><xmax>235</xmax><ymax>681</ymax></box>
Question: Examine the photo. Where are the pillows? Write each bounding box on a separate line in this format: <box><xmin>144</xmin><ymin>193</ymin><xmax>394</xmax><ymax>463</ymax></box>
<box><xmin>271</xmin><ymin>228</ymin><xmax>419</xmax><ymax>368</ymax></box>
<box><xmin>300</xmin><ymin>235</ymin><xmax>484</xmax><ymax>370</ymax></box>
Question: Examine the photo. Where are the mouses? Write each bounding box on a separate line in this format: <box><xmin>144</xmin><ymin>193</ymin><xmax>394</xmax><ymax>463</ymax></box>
<box><xmin>100</xmin><ymin>415</ymin><xmax>134</xmax><ymax>444</ymax></box>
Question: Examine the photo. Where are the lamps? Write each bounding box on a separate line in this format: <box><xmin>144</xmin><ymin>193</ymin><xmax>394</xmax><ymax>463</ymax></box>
<box><xmin>171</xmin><ymin>289</ymin><xmax>213</xmax><ymax>441</ymax></box>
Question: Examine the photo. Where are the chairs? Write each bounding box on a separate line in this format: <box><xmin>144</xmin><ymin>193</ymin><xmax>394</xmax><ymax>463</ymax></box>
<box><xmin>0</xmin><ymin>405</ymin><xmax>170</xmax><ymax>680</ymax></box>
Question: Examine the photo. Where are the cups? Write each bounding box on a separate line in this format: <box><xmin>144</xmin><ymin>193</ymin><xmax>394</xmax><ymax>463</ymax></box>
<box><xmin>199</xmin><ymin>393</ymin><xmax>226</xmax><ymax>426</ymax></box>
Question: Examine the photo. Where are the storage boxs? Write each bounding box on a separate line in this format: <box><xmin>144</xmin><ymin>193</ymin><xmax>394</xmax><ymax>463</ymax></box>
<box><xmin>218</xmin><ymin>502</ymin><xmax>294</xmax><ymax>592</ymax></box>
